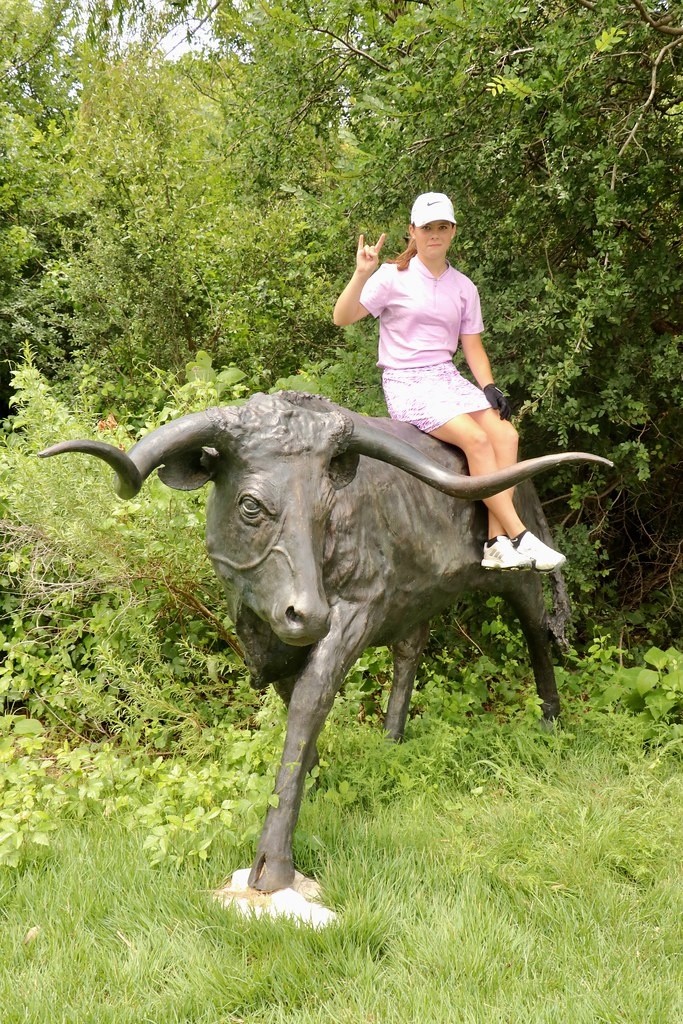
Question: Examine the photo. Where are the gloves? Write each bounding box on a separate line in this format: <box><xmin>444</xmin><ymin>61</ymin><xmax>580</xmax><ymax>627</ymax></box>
<box><xmin>485</xmin><ymin>384</ymin><xmax>512</xmax><ymax>422</ymax></box>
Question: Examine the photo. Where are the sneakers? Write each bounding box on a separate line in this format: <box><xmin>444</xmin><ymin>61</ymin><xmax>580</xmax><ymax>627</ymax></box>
<box><xmin>480</xmin><ymin>536</ymin><xmax>536</xmax><ymax>572</ymax></box>
<box><xmin>514</xmin><ymin>530</ymin><xmax>567</xmax><ymax>575</ymax></box>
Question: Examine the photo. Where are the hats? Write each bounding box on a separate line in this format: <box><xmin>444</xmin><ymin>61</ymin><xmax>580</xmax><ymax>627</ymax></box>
<box><xmin>410</xmin><ymin>191</ymin><xmax>457</xmax><ymax>227</ymax></box>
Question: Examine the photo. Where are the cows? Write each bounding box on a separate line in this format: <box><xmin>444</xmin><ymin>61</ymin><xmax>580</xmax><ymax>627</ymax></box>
<box><xmin>36</xmin><ymin>390</ymin><xmax>617</xmax><ymax>899</ymax></box>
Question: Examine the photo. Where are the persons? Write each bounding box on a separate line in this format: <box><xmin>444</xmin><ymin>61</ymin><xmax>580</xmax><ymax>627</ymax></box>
<box><xmin>334</xmin><ymin>192</ymin><xmax>565</xmax><ymax>574</ymax></box>
